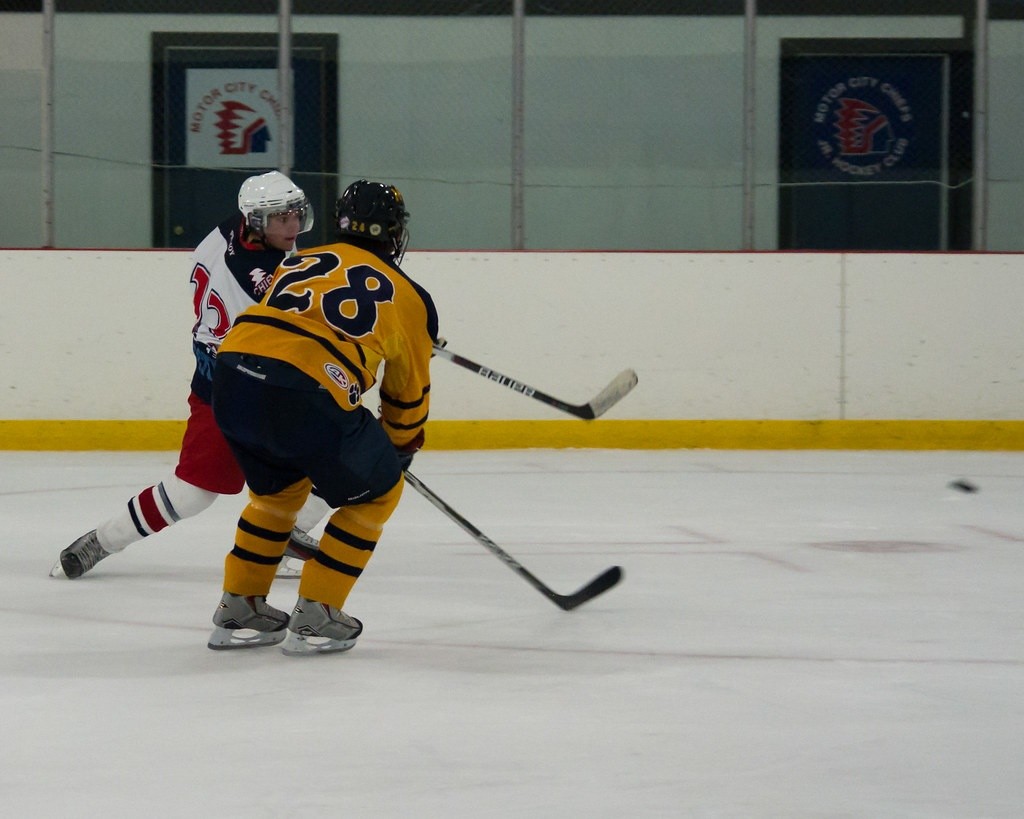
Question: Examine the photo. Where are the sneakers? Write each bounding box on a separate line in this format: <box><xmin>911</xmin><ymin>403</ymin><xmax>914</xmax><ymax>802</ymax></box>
<box><xmin>273</xmin><ymin>524</ymin><xmax>320</xmax><ymax>577</ymax></box>
<box><xmin>281</xmin><ymin>594</ymin><xmax>364</xmax><ymax>656</ymax></box>
<box><xmin>207</xmin><ymin>591</ymin><xmax>291</xmax><ymax>650</ymax></box>
<box><xmin>49</xmin><ymin>529</ymin><xmax>113</xmax><ymax>580</ymax></box>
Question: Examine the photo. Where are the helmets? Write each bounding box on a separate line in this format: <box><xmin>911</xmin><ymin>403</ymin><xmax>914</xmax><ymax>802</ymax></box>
<box><xmin>238</xmin><ymin>170</ymin><xmax>306</xmax><ymax>235</ymax></box>
<box><xmin>333</xmin><ymin>178</ymin><xmax>410</xmax><ymax>245</ymax></box>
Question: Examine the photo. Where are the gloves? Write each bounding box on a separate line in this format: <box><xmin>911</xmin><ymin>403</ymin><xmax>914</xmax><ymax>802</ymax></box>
<box><xmin>376</xmin><ymin>416</ymin><xmax>426</xmax><ymax>474</ymax></box>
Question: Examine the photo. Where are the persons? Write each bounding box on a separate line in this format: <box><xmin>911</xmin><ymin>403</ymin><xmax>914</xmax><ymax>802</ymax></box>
<box><xmin>210</xmin><ymin>175</ymin><xmax>440</xmax><ymax>660</ymax></box>
<box><xmin>36</xmin><ymin>167</ymin><xmax>449</xmax><ymax>582</ymax></box>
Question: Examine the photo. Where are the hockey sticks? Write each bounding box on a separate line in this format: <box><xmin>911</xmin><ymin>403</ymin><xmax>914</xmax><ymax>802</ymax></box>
<box><xmin>403</xmin><ymin>471</ymin><xmax>624</xmax><ymax>612</ymax></box>
<box><xmin>431</xmin><ymin>345</ymin><xmax>639</xmax><ymax>421</ymax></box>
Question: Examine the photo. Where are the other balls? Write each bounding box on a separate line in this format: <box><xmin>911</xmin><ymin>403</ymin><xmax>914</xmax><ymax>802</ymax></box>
<box><xmin>950</xmin><ymin>480</ymin><xmax>976</xmax><ymax>495</ymax></box>
